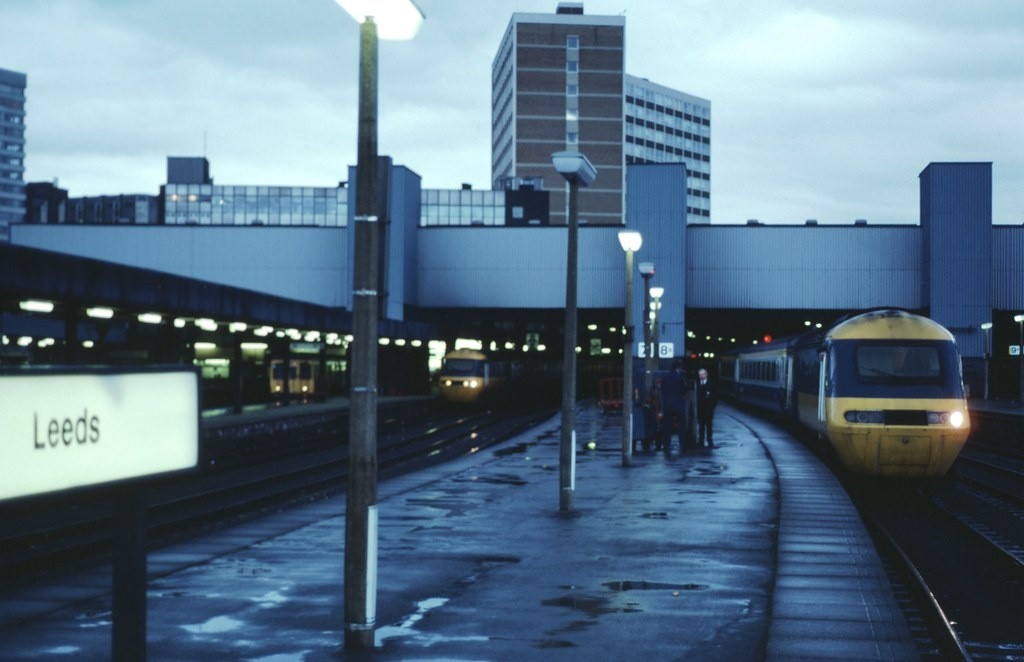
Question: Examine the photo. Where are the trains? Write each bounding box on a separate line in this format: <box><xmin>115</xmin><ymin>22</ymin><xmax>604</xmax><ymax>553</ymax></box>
<box><xmin>716</xmin><ymin>309</ymin><xmax>971</xmax><ymax>500</ymax></box>
<box><xmin>440</xmin><ymin>348</ymin><xmax>624</xmax><ymax>404</ymax></box>
<box><xmin>269</xmin><ymin>360</ymin><xmax>350</xmax><ymax>408</ymax></box>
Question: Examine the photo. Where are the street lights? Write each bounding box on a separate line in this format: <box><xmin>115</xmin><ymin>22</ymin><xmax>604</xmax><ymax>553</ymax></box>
<box><xmin>638</xmin><ymin>260</ymin><xmax>654</xmax><ymax>449</ymax></box>
<box><xmin>334</xmin><ymin>0</ymin><xmax>425</xmax><ymax>652</ymax></box>
<box><xmin>650</xmin><ymin>286</ymin><xmax>668</xmax><ymax>372</ymax></box>
<box><xmin>551</xmin><ymin>148</ymin><xmax>597</xmax><ymax>517</ymax></box>
<box><xmin>616</xmin><ymin>230</ymin><xmax>642</xmax><ymax>467</ymax></box>
<box><xmin>1014</xmin><ymin>315</ymin><xmax>1024</xmax><ymax>404</ymax></box>
<box><xmin>981</xmin><ymin>323</ymin><xmax>993</xmax><ymax>401</ymax></box>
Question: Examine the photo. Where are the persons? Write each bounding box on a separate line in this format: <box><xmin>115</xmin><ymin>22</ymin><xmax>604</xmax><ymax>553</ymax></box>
<box><xmin>661</xmin><ymin>359</ymin><xmax>691</xmax><ymax>452</ymax></box>
<box><xmin>698</xmin><ymin>368</ymin><xmax>719</xmax><ymax>448</ymax></box>
